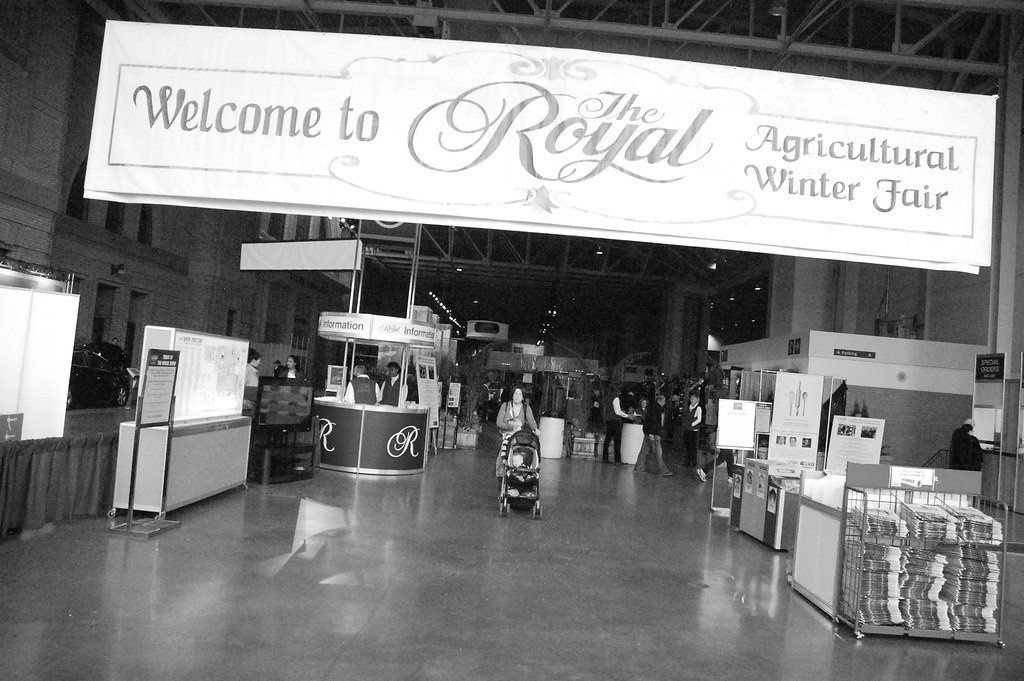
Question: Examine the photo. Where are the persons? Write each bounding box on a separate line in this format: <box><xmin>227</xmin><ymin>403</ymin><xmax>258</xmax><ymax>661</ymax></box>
<box><xmin>867</xmin><ymin>428</ymin><xmax>874</xmax><ymax>438</ymax></box>
<box><xmin>243</xmin><ymin>348</ymin><xmax>263</xmax><ymax>414</ymax></box>
<box><xmin>684</xmin><ymin>394</ymin><xmax>735</xmax><ymax>484</ymax></box>
<box><xmin>481</xmin><ymin>380</ymin><xmax>492</xmax><ymax>423</ymax></box>
<box><xmin>273</xmin><ymin>354</ymin><xmax>304</xmax><ymax>380</ymax></box>
<box><xmin>840</xmin><ymin>425</ymin><xmax>852</xmax><ymax>436</ymax></box>
<box><xmin>112</xmin><ymin>337</ymin><xmax>119</xmax><ymax>345</ymax></box>
<box><xmin>496</xmin><ymin>384</ymin><xmax>541</xmax><ymax>500</ymax></box>
<box><xmin>769</xmin><ymin>488</ymin><xmax>777</xmax><ymax>513</ymax></box>
<box><xmin>950</xmin><ymin>419</ymin><xmax>983</xmax><ymax>471</ymax></box>
<box><xmin>344</xmin><ymin>361</ymin><xmax>406</xmax><ymax>407</ymax></box>
<box><xmin>789</xmin><ymin>437</ymin><xmax>797</xmax><ymax>447</ymax></box>
<box><xmin>602</xmin><ymin>388</ymin><xmax>674</xmax><ymax>476</ymax></box>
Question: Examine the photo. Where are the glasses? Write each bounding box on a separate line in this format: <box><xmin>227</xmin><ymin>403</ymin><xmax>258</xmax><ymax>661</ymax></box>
<box><xmin>513</xmin><ymin>393</ymin><xmax>522</xmax><ymax>397</ymax></box>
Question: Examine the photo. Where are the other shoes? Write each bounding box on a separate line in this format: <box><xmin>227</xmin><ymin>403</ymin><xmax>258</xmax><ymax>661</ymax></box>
<box><xmin>616</xmin><ymin>461</ymin><xmax>627</xmax><ymax>465</ymax></box>
<box><xmin>602</xmin><ymin>460</ymin><xmax>613</xmax><ymax>464</ymax></box>
<box><xmin>684</xmin><ymin>464</ymin><xmax>690</xmax><ymax>466</ymax></box>
<box><xmin>659</xmin><ymin>472</ymin><xmax>672</xmax><ymax>476</ymax></box>
<box><xmin>691</xmin><ymin>464</ymin><xmax>697</xmax><ymax>467</ymax></box>
<box><xmin>634</xmin><ymin>468</ymin><xmax>647</xmax><ymax>475</ymax></box>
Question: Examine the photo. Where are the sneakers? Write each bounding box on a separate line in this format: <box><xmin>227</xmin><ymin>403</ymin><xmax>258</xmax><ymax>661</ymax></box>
<box><xmin>728</xmin><ymin>477</ymin><xmax>732</xmax><ymax>483</ymax></box>
<box><xmin>697</xmin><ymin>468</ymin><xmax>707</xmax><ymax>481</ymax></box>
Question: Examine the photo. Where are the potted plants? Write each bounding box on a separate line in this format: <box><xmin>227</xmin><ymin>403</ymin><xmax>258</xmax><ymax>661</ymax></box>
<box><xmin>436</xmin><ymin>347</ymin><xmax>488</xmax><ymax>450</ymax></box>
<box><xmin>567</xmin><ymin>359</ymin><xmax>614</xmax><ymax>460</ymax></box>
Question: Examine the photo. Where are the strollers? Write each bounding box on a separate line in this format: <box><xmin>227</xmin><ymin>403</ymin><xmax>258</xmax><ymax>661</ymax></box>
<box><xmin>498</xmin><ymin>429</ymin><xmax>542</xmax><ymax>520</ymax></box>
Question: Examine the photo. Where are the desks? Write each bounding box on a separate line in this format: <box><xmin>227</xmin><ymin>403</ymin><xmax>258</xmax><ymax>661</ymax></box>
<box><xmin>791</xmin><ymin>469</ymin><xmax>973</xmax><ymax>621</ymax></box>
<box><xmin>251</xmin><ymin>442</ymin><xmax>317</xmax><ymax>485</ymax></box>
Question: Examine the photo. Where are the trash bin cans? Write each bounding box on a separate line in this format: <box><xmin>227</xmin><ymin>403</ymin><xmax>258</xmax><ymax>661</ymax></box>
<box><xmin>729</xmin><ymin>456</ymin><xmax>802</xmax><ymax>551</ymax></box>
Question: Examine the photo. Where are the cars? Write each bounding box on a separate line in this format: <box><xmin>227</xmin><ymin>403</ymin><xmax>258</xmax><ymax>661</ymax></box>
<box><xmin>72</xmin><ymin>342</ymin><xmax>130</xmax><ymax>376</ymax></box>
<box><xmin>66</xmin><ymin>349</ymin><xmax>130</xmax><ymax>410</ymax></box>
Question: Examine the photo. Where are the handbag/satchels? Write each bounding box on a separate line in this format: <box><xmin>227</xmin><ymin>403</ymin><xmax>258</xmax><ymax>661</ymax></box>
<box><xmin>966</xmin><ymin>439</ymin><xmax>983</xmax><ymax>467</ymax></box>
